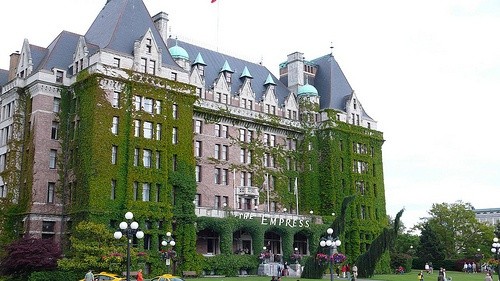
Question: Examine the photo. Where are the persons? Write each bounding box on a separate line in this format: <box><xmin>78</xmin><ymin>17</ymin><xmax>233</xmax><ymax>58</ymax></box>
<box><xmin>484</xmin><ymin>272</ymin><xmax>492</xmax><ymax>281</ymax></box>
<box><xmin>419</xmin><ymin>270</ymin><xmax>424</xmax><ymax>281</ymax></box>
<box><xmin>85</xmin><ymin>270</ymin><xmax>94</xmax><ymax>281</ymax></box>
<box><xmin>438</xmin><ymin>266</ymin><xmax>452</xmax><ymax>281</ymax></box>
<box><xmin>485</xmin><ymin>264</ymin><xmax>487</xmax><ymax>272</ymax></box>
<box><xmin>265</xmin><ymin>245</ymin><xmax>271</xmax><ymax>253</ymax></box>
<box><xmin>425</xmin><ymin>261</ymin><xmax>433</xmax><ymax>275</ymax></box>
<box><xmin>137</xmin><ymin>269</ymin><xmax>143</xmax><ymax>281</ymax></box>
<box><xmin>336</xmin><ymin>266</ymin><xmax>340</xmax><ymax>278</ymax></box>
<box><xmin>472</xmin><ymin>262</ymin><xmax>477</xmax><ymax>273</ymax></box>
<box><xmin>341</xmin><ymin>264</ymin><xmax>350</xmax><ymax>278</ymax></box>
<box><xmin>277</xmin><ymin>262</ymin><xmax>281</xmax><ymax>277</ymax></box>
<box><xmin>351</xmin><ymin>264</ymin><xmax>358</xmax><ymax>281</ymax></box>
<box><xmin>284</xmin><ymin>262</ymin><xmax>288</xmax><ymax>276</ymax></box>
<box><xmin>274</xmin><ymin>254</ymin><xmax>281</xmax><ymax>264</ymax></box>
<box><xmin>464</xmin><ymin>262</ymin><xmax>467</xmax><ymax>273</ymax></box>
<box><xmin>271</xmin><ymin>276</ymin><xmax>280</xmax><ymax>281</ymax></box>
<box><xmin>467</xmin><ymin>262</ymin><xmax>472</xmax><ymax>273</ymax></box>
<box><xmin>293</xmin><ymin>262</ymin><xmax>298</xmax><ymax>276</ymax></box>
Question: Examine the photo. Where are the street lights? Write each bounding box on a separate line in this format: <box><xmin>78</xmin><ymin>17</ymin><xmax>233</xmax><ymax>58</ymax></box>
<box><xmin>490</xmin><ymin>237</ymin><xmax>500</xmax><ymax>261</ymax></box>
<box><xmin>320</xmin><ymin>227</ymin><xmax>342</xmax><ymax>281</ymax></box>
<box><xmin>161</xmin><ymin>231</ymin><xmax>176</xmax><ymax>274</ymax></box>
<box><xmin>114</xmin><ymin>212</ymin><xmax>144</xmax><ymax>281</ymax></box>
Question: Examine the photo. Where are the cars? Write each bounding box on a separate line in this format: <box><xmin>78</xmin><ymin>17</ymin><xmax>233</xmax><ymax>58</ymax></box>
<box><xmin>150</xmin><ymin>273</ymin><xmax>186</xmax><ymax>281</ymax></box>
<box><xmin>79</xmin><ymin>271</ymin><xmax>138</xmax><ymax>281</ymax></box>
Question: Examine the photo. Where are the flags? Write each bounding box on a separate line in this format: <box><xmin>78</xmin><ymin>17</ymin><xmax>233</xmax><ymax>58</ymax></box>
<box><xmin>211</xmin><ymin>0</ymin><xmax>216</xmax><ymax>3</ymax></box>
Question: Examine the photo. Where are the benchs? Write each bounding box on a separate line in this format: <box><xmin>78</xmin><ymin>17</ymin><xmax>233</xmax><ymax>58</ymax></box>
<box><xmin>182</xmin><ymin>270</ymin><xmax>199</xmax><ymax>278</ymax></box>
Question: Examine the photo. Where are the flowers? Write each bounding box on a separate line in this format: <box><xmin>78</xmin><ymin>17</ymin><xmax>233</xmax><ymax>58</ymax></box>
<box><xmin>314</xmin><ymin>252</ymin><xmax>329</xmax><ymax>267</ymax></box>
<box><xmin>330</xmin><ymin>252</ymin><xmax>347</xmax><ymax>264</ymax></box>
